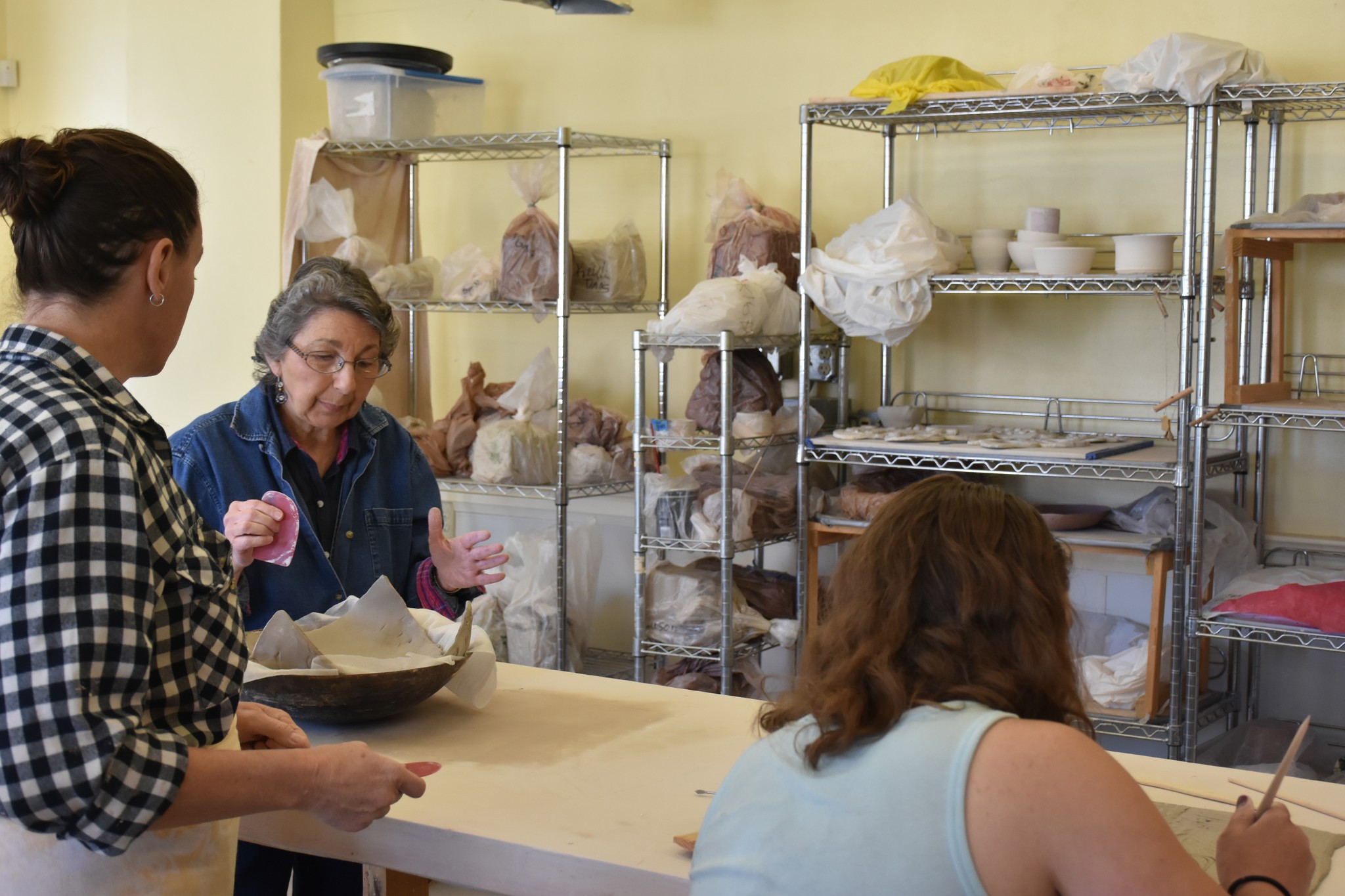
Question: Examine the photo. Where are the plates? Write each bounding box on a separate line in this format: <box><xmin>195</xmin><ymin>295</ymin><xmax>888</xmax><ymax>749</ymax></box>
<box><xmin>1034</xmin><ymin>503</ymin><xmax>1110</xmax><ymax>530</ymax></box>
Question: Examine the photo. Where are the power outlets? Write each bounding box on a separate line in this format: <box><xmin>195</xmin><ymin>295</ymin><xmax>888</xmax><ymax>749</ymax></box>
<box><xmin>810</xmin><ymin>345</ymin><xmax>836</xmax><ymax>382</ymax></box>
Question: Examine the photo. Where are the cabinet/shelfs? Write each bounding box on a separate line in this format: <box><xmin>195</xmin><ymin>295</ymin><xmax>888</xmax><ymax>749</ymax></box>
<box><xmin>297</xmin><ymin>126</ymin><xmax>671</xmax><ymax>681</ymax></box>
<box><xmin>796</xmin><ymin>89</ymin><xmax>1253</xmax><ymax>759</ymax></box>
<box><xmin>1186</xmin><ymin>82</ymin><xmax>1345</xmax><ymax>762</ymax></box>
<box><xmin>633</xmin><ymin>330</ymin><xmax>847</xmax><ymax>695</ymax></box>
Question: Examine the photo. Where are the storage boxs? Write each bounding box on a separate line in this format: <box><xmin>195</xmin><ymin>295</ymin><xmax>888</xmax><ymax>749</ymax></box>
<box><xmin>318</xmin><ymin>63</ymin><xmax>486</xmax><ymax>140</ymax></box>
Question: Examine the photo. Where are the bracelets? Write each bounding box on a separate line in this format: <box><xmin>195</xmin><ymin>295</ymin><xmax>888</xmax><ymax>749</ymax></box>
<box><xmin>1227</xmin><ymin>874</ymin><xmax>1290</xmax><ymax>895</ymax></box>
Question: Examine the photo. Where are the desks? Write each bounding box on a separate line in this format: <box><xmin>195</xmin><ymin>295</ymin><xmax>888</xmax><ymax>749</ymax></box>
<box><xmin>236</xmin><ymin>632</ymin><xmax>1345</xmax><ymax>896</ymax></box>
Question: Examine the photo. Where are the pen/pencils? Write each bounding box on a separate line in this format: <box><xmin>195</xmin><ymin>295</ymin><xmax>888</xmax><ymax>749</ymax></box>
<box><xmin>1251</xmin><ymin>714</ymin><xmax>1313</xmax><ymax>828</ymax></box>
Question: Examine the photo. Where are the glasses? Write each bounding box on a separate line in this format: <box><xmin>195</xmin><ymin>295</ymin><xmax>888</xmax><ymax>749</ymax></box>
<box><xmin>284</xmin><ymin>339</ymin><xmax>392</xmax><ymax>379</ymax></box>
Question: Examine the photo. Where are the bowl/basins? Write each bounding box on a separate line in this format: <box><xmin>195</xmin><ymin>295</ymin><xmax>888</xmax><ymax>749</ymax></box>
<box><xmin>878</xmin><ymin>406</ymin><xmax>926</xmax><ymax>429</ymax></box>
<box><xmin>1111</xmin><ymin>235</ymin><xmax>1178</xmax><ymax>273</ymax></box>
<box><xmin>1006</xmin><ymin>241</ymin><xmax>1095</xmax><ymax>277</ymax></box>
<box><xmin>646</xmin><ymin>420</ymin><xmax>697</xmax><ymax>453</ymax></box>
<box><xmin>238</xmin><ymin>652</ymin><xmax>476</xmax><ymax>721</ymax></box>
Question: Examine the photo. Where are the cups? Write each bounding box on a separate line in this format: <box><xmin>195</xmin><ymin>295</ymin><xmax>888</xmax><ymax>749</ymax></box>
<box><xmin>1017</xmin><ymin>206</ymin><xmax>1066</xmax><ymax>242</ymax></box>
<box><xmin>970</xmin><ymin>229</ymin><xmax>1013</xmax><ymax>275</ymax></box>
<box><xmin>655</xmin><ymin>487</ymin><xmax>699</xmax><ymax>545</ymax></box>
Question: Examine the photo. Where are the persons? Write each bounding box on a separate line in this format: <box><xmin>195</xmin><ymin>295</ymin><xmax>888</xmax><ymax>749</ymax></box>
<box><xmin>166</xmin><ymin>257</ymin><xmax>509</xmax><ymax>896</ymax></box>
<box><xmin>1</xmin><ymin>126</ymin><xmax>426</xmax><ymax>896</ymax></box>
<box><xmin>685</xmin><ymin>475</ymin><xmax>1317</xmax><ymax>896</ymax></box>
